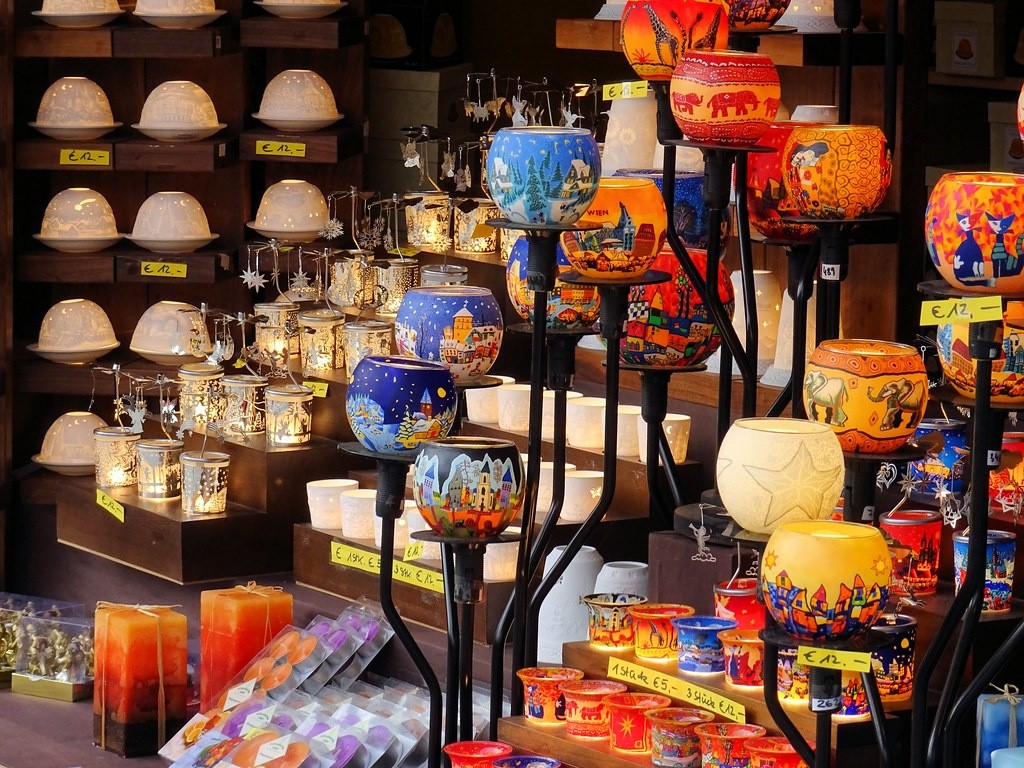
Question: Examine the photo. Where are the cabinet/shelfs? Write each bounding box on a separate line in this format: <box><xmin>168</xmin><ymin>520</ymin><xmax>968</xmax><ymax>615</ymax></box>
<box><xmin>1</xmin><ymin>1</ymin><xmax>362</xmax><ymax>506</ymax></box>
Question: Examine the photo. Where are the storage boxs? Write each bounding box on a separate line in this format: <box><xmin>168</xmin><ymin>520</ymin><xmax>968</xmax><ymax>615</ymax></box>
<box><xmin>0</xmin><ymin>591</ymin><xmax>95</xmax><ymax>702</ymax></box>
<box><xmin>976</xmin><ymin>681</ymin><xmax>1023</xmax><ymax>767</ymax></box>
<box><xmin>934</xmin><ymin>1</ymin><xmax>1015</xmax><ymax>78</ymax></box>
<box><xmin>158</xmin><ymin>595</ymin><xmax>512</xmax><ymax>768</ymax></box>
<box><xmin>365</xmin><ymin>62</ymin><xmax>476</xmax><ymax>190</ymax></box>
<box><xmin>988</xmin><ymin>101</ymin><xmax>1024</xmax><ymax>173</ymax></box>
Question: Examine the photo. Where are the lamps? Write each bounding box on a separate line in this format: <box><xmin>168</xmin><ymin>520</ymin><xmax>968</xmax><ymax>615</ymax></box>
<box><xmin>306</xmin><ymin>0</ymin><xmax>840</xmax><ymax>582</ymax></box>
<box><xmin>32</xmin><ymin>1</ymin><xmax>344</xmax><ymax>476</ymax></box>
<box><xmin>346</xmin><ymin>0</ymin><xmax>892</xmax><ymax>768</ymax></box>
<box><xmin>93</xmin><ymin>69</ymin><xmax>602</xmax><ymax>515</ymax></box>
<box><xmin>445</xmin><ymin>171</ymin><xmax>1024</xmax><ymax>768</ymax></box>
